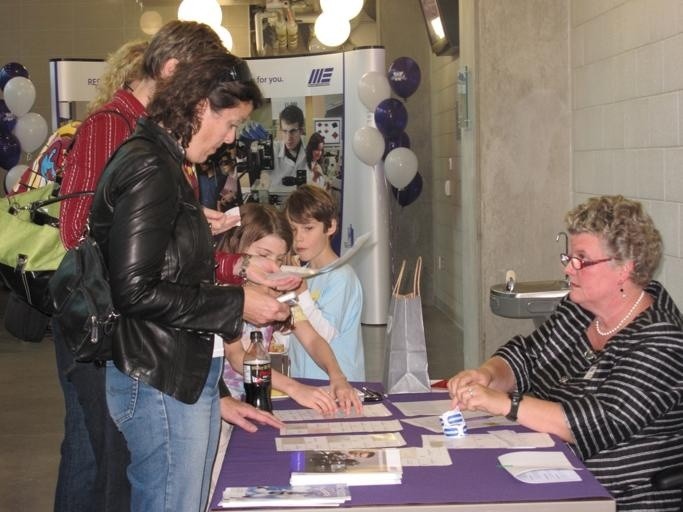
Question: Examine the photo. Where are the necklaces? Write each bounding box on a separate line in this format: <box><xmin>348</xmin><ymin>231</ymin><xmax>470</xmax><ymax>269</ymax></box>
<box><xmin>595</xmin><ymin>292</ymin><xmax>644</xmax><ymax>335</ymax></box>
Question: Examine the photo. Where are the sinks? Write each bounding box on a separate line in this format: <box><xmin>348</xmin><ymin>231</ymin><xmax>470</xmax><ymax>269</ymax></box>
<box><xmin>490</xmin><ymin>279</ymin><xmax>570</xmax><ymax>318</ymax></box>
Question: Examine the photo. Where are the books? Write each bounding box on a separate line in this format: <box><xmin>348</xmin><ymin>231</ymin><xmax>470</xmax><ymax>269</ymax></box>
<box><xmin>218</xmin><ymin>449</ymin><xmax>403</xmax><ymax>507</ymax></box>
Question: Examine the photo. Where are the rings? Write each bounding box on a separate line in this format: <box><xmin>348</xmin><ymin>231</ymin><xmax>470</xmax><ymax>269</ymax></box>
<box><xmin>470</xmin><ymin>391</ymin><xmax>473</xmax><ymax>396</ymax></box>
<box><xmin>469</xmin><ymin>389</ymin><xmax>471</xmax><ymax>392</ymax></box>
<box><xmin>255</xmin><ymin>406</ymin><xmax>262</xmax><ymax>411</ymax></box>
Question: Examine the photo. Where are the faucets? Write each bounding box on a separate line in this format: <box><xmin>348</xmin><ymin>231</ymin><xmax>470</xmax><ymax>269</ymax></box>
<box><xmin>556</xmin><ymin>231</ymin><xmax>571</xmax><ymax>290</ymax></box>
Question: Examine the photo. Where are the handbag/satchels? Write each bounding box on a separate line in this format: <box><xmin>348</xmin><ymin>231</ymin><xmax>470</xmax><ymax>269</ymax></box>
<box><xmin>1</xmin><ymin>183</ymin><xmax>74</xmax><ymax>274</ymax></box>
<box><xmin>383</xmin><ymin>256</ymin><xmax>433</xmax><ymax>396</ymax></box>
<box><xmin>47</xmin><ymin>237</ymin><xmax>119</xmax><ymax>365</ymax></box>
<box><xmin>4</xmin><ymin>292</ymin><xmax>51</xmax><ymax>343</ymax></box>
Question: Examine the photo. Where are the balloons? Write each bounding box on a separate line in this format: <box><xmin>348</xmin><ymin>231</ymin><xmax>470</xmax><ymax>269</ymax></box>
<box><xmin>1</xmin><ymin>63</ymin><xmax>48</xmax><ymax>197</ymax></box>
<box><xmin>352</xmin><ymin>56</ymin><xmax>422</xmax><ymax>207</ymax></box>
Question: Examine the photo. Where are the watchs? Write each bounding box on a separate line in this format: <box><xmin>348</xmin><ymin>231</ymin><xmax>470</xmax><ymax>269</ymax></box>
<box><xmin>504</xmin><ymin>388</ymin><xmax>522</xmax><ymax>420</ymax></box>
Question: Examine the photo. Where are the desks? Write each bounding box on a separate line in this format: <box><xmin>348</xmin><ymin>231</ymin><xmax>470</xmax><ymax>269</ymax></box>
<box><xmin>203</xmin><ymin>375</ymin><xmax>617</xmax><ymax>512</ymax></box>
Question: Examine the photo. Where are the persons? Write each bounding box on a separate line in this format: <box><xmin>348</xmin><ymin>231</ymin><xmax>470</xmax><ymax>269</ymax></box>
<box><xmin>196</xmin><ymin>155</ymin><xmax>223</xmax><ymax>209</ymax></box>
<box><xmin>54</xmin><ymin>22</ymin><xmax>304</xmax><ymax>512</ymax></box>
<box><xmin>267</xmin><ymin>104</ymin><xmax>305</xmax><ymax>196</ymax></box>
<box><xmin>66</xmin><ymin>40</ymin><xmax>149</xmax><ymax>198</ymax></box>
<box><xmin>279</xmin><ymin>183</ymin><xmax>364</xmax><ymax>380</ymax></box>
<box><xmin>99</xmin><ymin>56</ymin><xmax>290</xmax><ymax>511</ymax></box>
<box><xmin>447</xmin><ymin>196</ymin><xmax>683</xmax><ymax>512</ymax></box>
<box><xmin>217</xmin><ymin>150</ymin><xmax>238</xmax><ymax>193</ymax></box>
<box><xmin>206</xmin><ymin>202</ymin><xmax>364</xmax><ymax>512</ymax></box>
<box><xmin>304</xmin><ymin>132</ymin><xmax>329</xmax><ymax>190</ymax></box>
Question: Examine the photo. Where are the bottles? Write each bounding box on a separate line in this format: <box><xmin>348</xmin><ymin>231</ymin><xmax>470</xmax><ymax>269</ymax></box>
<box><xmin>244</xmin><ymin>330</ymin><xmax>272</xmax><ymax>416</ymax></box>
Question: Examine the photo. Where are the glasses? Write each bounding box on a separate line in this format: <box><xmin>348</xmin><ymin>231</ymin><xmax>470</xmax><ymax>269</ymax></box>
<box><xmin>279</xmin><ymin>126</ymin><xmax>300</xmax><ymax>136</ymax></box>
<box><xmin>559</xmin><ymin>252</ymin><xmax>621</xmax><ymax>269</ymax></box>
<box><xmin>207</xmin><ymin>61</ymin><xmax>254</xmax><ymax>98</ymax></box>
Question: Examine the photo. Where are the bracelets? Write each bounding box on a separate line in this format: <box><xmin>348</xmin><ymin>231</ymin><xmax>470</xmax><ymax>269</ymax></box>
<box><xmin>239</xmin><ymin>253</ymin><xmax>252</xmax><ymax>283</ymax></box>
